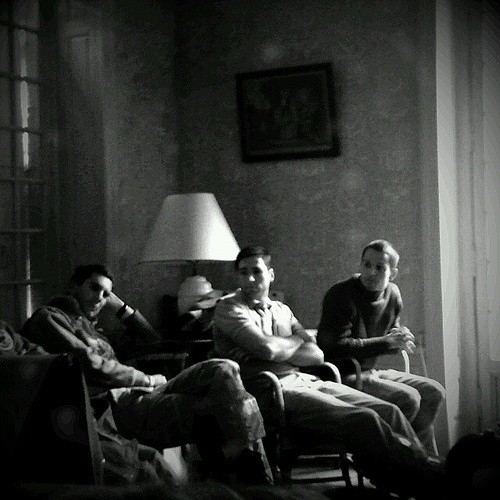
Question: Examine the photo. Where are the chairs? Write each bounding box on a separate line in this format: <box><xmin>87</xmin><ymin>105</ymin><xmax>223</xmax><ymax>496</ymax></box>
<box><xmin>0</xmin><ymin>313</ymin><xmax>427</xmax><ymax>500</ymax></box>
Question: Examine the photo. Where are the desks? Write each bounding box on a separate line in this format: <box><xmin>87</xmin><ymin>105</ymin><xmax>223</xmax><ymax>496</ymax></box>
<box><xmin>161</xmin><ymin>290</ymin><xmax>288</xmax><ymax>371</ymax></box>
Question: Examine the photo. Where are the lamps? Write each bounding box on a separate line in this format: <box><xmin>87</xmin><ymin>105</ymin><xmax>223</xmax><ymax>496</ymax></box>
<box><xmin>135</xmin><ymin>191</ymin><xmax>240</xmax><ymax>315</ymax></box>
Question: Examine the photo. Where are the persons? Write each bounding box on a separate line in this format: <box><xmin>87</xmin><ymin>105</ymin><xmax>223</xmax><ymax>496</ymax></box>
<box><xmin>214</xmin><ymin>244</ymin><xmax>444</xmax><ymax>500</ymax></box>
<box><xmin>316</xmin><ymin>239</ymin><xmax>445</xmax><ymax>445</ymax></box>
<box><xmin>0</xmin><ymin>320</ymin><xmax>179</xmax><ymax>491</ymax></box>
<box><xmin>24</xmin><ymin>264</ymin><xmax>272</xmax><ymax>483</ymax></box>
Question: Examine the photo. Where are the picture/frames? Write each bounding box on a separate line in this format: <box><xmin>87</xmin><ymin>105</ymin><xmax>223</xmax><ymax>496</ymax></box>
<box><xmin>234</xmin><ymin>61</ymin><xmax>342</xmax><ymax>165</ymax></box>
